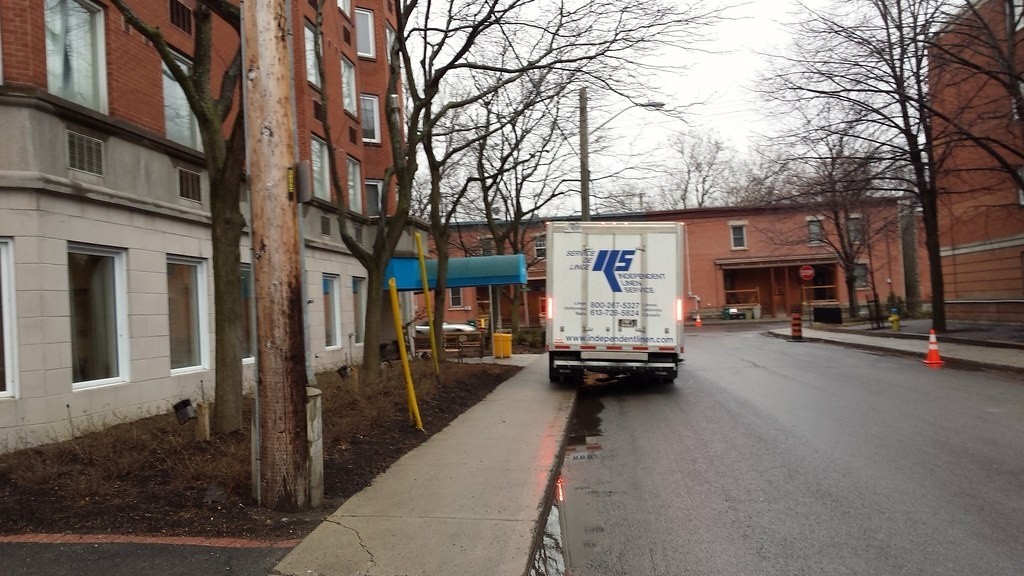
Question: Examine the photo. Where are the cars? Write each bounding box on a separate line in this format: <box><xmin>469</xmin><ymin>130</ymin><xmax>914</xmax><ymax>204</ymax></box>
<box><xmin>414</xmin><ymin>320</ymin><xmax>479</xmax><ymax>341</ymax></box>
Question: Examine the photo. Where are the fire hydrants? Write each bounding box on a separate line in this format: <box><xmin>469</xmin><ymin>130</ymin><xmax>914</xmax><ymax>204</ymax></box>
<box><xmin>887</xmin><ymin>309</ymin><xmax>903</xmax><ymax>333</ymax></box>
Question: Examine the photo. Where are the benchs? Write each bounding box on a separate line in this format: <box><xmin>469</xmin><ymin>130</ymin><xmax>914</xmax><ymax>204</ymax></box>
<box><xmin>444</xmin><ymin>332</ymin><xmax>483</xmax><ymax>359</ymax></box>
<box><xmin>720</xmin><ymin>303</ymin><xmax>757</xmax><ymax>320</ymax></box>
<box><xmin>414</xmin><ymin>336</ymin><xmax>463</xmax><ymax>363</ymax></box>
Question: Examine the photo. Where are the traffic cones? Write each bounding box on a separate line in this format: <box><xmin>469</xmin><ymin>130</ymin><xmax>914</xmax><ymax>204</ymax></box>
<box><xmin>694</xmin><ymin>315</ymin><xmax>702</xmax><ymax>327</ymax></box>
<box><xmin>923</xmin><ymin>330</ymin><xmax>945</xmax><ymax>364</ymax></box>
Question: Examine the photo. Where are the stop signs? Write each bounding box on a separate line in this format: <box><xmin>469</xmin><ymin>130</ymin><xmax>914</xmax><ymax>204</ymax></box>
<box><xmin>799</xmin><ymin>266</ymin><xmax>816</xmax><ymax>281</ymax></box>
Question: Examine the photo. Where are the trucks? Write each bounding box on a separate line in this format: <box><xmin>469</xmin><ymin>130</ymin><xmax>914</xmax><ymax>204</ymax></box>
<box><xmin>545</xmin><ymin>221</ymin><xmax>687</xmax><ymax>384</ymax></box>
